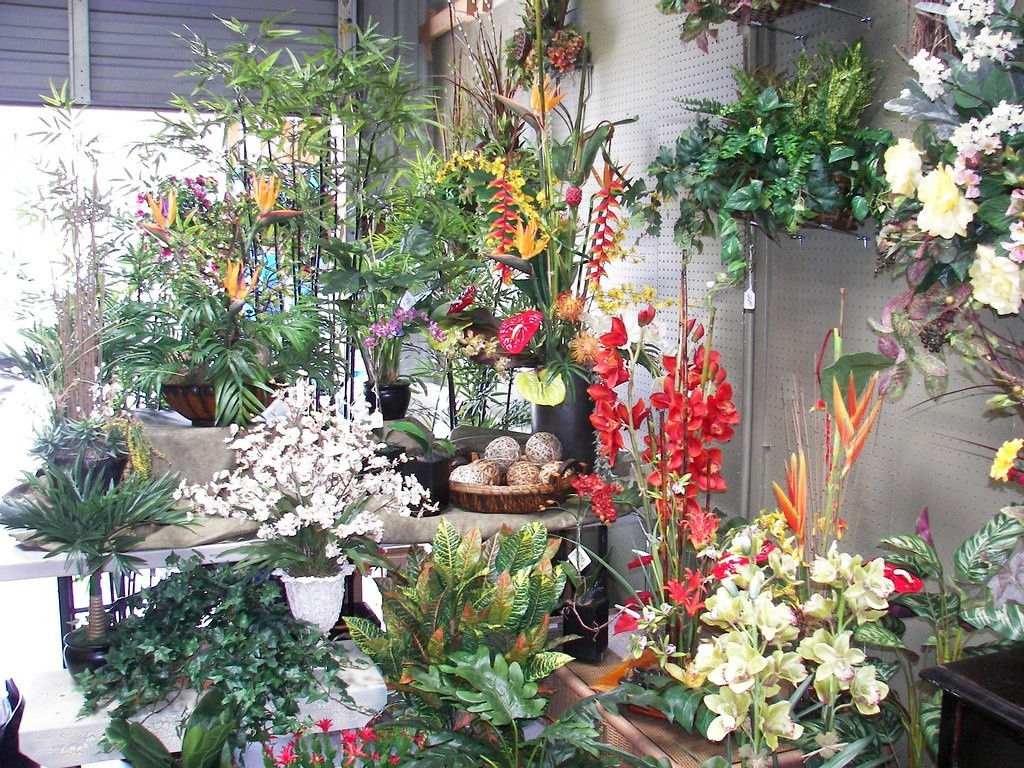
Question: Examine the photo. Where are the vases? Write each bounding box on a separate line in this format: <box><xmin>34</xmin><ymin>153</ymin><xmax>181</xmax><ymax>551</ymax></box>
<box><xmin>266</xmin><ymin>555</ymin><xmax>356</xmax><ymax>655</ymax></box>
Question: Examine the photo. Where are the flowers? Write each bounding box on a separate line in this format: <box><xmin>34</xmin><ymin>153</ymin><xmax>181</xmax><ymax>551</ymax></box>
<box><xmin>133</xmin><ymin>0</ymin><xmax>1024</xmax><ymax>768</ymax></box>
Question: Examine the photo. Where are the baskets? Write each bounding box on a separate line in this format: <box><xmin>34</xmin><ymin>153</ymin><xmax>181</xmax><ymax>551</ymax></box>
<box><xmin>447</xmin><ymin>459</ymin><xmax>587</xmax><ymax>514</ymax></box>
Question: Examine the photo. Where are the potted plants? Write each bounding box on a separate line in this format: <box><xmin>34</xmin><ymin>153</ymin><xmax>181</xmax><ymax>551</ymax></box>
<box><xmin>0</xmin><ymin>446</ymin><xmax>203</xmax><ymax>696</ymax></box>
<box><xmin>104</xmin><ymin>179</ymin><xmax>328</xmax><ymax>428</ymax></box>
<box><xmin>316</xmin><ymin>222</ymin><xmax>426</xmax><ymax>421</ymax></box>
